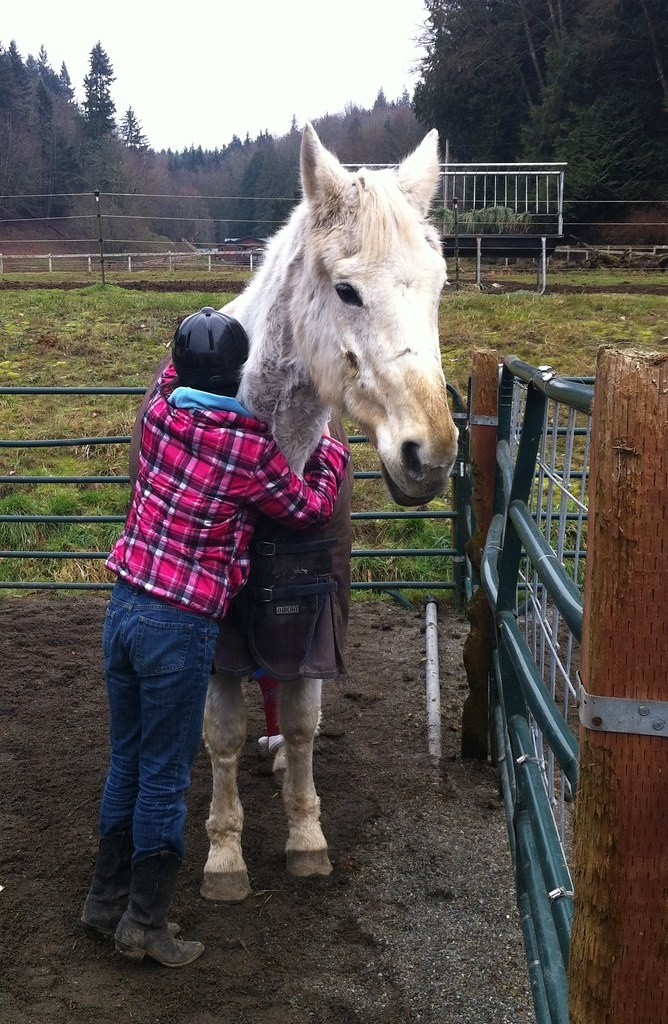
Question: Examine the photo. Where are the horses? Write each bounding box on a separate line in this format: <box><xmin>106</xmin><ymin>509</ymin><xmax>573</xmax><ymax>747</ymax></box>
<box><xmin>197</xmin><ymin>119</ymin><xmax>463</xmax><ymax>902</ymax></box>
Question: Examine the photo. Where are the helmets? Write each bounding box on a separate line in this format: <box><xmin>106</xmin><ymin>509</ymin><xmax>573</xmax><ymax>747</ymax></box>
<box><xmin>172</xmin><ymin>306</ymin><xmax>250</xmax><ymax>396</ymax></box>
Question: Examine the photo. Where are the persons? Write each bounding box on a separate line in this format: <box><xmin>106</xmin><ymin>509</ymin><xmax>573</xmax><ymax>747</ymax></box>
<box><xmin>80</xmin><ymin>306</ymin><xmax>351</xmax><ymax>966</ymax></box>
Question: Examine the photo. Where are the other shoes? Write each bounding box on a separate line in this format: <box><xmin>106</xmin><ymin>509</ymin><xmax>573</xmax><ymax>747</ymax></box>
<box><xmin>268</xmin><ymin>734</ymin><xmax>286</xmax><ymax>751</ymax></box>
<box><xmin>258</xmin><ymin>736</ymin><xmax>268</xmax><ymax>744</ymax></box>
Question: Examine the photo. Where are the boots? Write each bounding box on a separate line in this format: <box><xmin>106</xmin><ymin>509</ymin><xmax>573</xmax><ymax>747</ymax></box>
<box><xmin>115</xmin><ymin>850</ymin><xmax>205</xmax><ymax>968</ymax></box>
<box><xmin>81</xmin><ymin>831</ymin><xmax>181</xmax><ymax>943</ymax></box>
